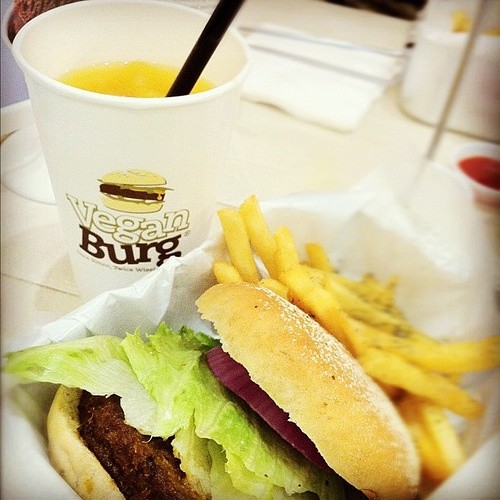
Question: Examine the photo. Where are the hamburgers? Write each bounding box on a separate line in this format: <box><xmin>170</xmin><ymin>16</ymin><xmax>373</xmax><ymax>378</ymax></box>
<box><xmin>1</xmin><ymin>279</ymin><xmax>420</xmax><ymax>500</ymax></box>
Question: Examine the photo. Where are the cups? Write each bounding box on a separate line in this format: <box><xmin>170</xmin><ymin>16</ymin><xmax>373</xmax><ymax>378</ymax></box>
<box><xmin>13</xmin><ymin>0</ymin><xmax>249</xmax><ymax>303</ymax></box>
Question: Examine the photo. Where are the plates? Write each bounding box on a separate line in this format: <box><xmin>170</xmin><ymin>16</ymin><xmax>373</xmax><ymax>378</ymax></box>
<box><xmin>0</xmin><ymin>86</ymin><xmax>500</xmax><ymax>500</ymax></box>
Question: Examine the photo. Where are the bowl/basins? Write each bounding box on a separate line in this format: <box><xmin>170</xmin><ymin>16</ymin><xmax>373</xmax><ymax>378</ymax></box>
<box><xmin>455</xmin><ymin>144</ymin><xmax>500</xmax><ymax>203</ymax></box>
<box><xmin>1</xmin><ymin>0</ymin><xmax>13</xmax><ymax>52</ymax></box>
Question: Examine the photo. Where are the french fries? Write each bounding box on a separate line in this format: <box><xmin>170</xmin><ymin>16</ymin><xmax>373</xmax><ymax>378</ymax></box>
<box><xmin>212</xmin><ymin>194</ymin><xmax>500</xmax><ymax>483</ymax></box>
